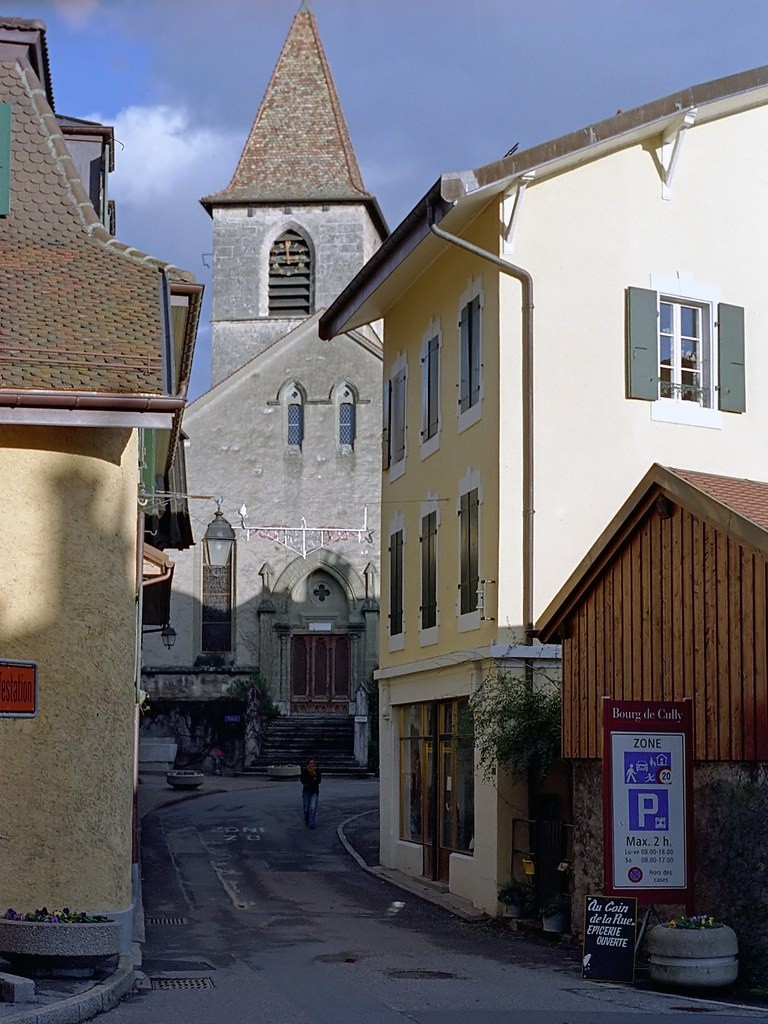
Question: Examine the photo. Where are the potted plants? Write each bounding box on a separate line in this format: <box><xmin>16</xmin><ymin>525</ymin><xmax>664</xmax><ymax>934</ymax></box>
<box><xmin>497</xmin><ymin>879</ymin><xmax>531</xmax><ymax>917</ymax></box>
<box><xmin>537</xmin><ymin>897</ymin><xmax>571</xmax><ymax>933</ymax></box>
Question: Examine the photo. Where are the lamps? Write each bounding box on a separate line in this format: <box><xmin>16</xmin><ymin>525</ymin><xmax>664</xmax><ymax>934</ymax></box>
<box><xmin>135</xmin><ymin>481</ymin><xmax>239</xmax><ymax>598</ymax></box>
<box><xmin>142</xmin><ymin>623</ymin><xmax>177</xmax><ymax>650</ymax></box>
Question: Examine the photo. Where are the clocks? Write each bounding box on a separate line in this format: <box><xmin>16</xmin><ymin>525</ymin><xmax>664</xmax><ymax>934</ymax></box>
<box><xmin>269</xmin><ymin>240</ymin><xmax>309</xmax><ymax>276</ymax></box>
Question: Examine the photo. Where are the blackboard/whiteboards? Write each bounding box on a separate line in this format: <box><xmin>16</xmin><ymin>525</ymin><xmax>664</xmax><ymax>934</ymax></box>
<box><xmin>582</xmin><ymin>895</ymin><xmax>637</xmax><ymax>984</ymax></box>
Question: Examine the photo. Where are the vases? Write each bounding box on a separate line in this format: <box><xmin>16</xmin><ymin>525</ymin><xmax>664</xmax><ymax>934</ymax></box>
<box><xmin>267</xmin><ymin>766</ymin><xmax>301</xmax><ymax>781</ymax></box>
<box><xmin>0</xmin><ymin>917</ymin><xmax>121</xmax><ymax>978</ymax></box>
<box><xmin>167</xmin><ymin>772</ymin><xmax>205</xmax><ymax>788</ymax></box>
<box><xmin>647</xmin><ymin>923</ymin><xmax>739</xmax><ymax>987</ymax></box>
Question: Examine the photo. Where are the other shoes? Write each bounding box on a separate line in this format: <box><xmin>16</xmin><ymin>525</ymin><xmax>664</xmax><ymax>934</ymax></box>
<box><xmin>309</xmin><ymin>825</ymin><xmax>316</xmax><ymax>830</ymax></box>
<box><xmin>305</xmin><ymin>821</ymin><xmax>309</xmax><ymax>827</ymax></box>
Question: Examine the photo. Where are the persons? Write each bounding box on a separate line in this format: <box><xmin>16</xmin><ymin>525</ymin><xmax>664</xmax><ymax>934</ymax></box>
<box><xmin>300</xmin><ymin>758</ymin><xmax>321</xmax><ymax>829</ymax></box>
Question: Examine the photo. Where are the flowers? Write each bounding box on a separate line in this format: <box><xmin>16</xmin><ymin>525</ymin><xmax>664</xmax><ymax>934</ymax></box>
<box><xmin>2</xmin><ymin>907</ymin><xmax>108</xmax><ymax>923</ymax></box>
<box><xmin>669</xmin><ymin>915</ymin><xmax>723</xmax><ymax>930</ymax></box>
